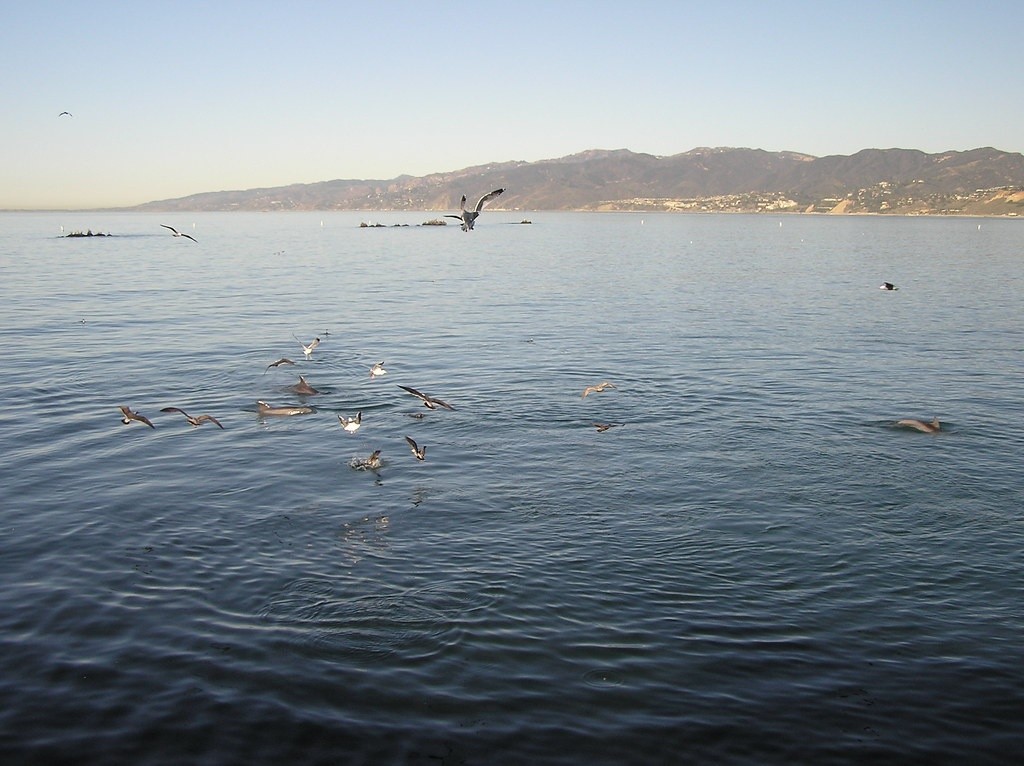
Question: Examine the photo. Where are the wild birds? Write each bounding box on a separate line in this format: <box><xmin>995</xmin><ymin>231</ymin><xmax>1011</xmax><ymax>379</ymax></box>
<box><xmin>159</xmin><ymin>406</ymin><xmax>226</xmax><ymax>431</ymax></box>
<box><xmin>254</xmin><ymin>327</ymin><xmax>332</xmax><ymax>417</ymax></box>
<box><xmin>337</xmin><ymin>411</ymin><xmax>362</xmax><ymax>435</ymax></box>
<box><xmin>58</xmin><ymin>112</ymin><xmax>72</xmax><ymax>118</ymax></box>
<box><xmin>592</xmin><ymin>422</ymin><xmax>618</xmax><ymax>433</ymax></box>
<box><xmin>880</xmin><ymin>282</ymin><xmax>900</xmax><ymax>291</ymax></box>
<box><xmin>160</xmin><ymin>223</ymin><xmax>199</xmax><ymax>245</ymax></box>
<box><xmin>582</xmin><ymin>381</ymin><xmax>617</xmax><ymax>400</ymax></box>
<box><xmin>443</xmin><ymin>187</ymin><xmax>507</xmax><ymax>233</ymax></box>
<box><xmin>396</xmin><ymin>384</ymin><xmax>459</xmax><ymax>464</ymax></box>
<box><xmin>347</xmin><ymin>449</ymin><xmax>385</xmax><ymax>477</ymax></box>
<box><xmin>116</xmin><ymin>405</ymin><xmax>156</xmax><ymax>430</ymax></box>
<box><xmin>897</xmin><ymin>415</ymin><xmax>943</xmax><ymax>435</ymax></box>
<box><xmin>369</xmin><ymin>361</ymin><xmax>391</xmax><ymax>378</ymax></box>
